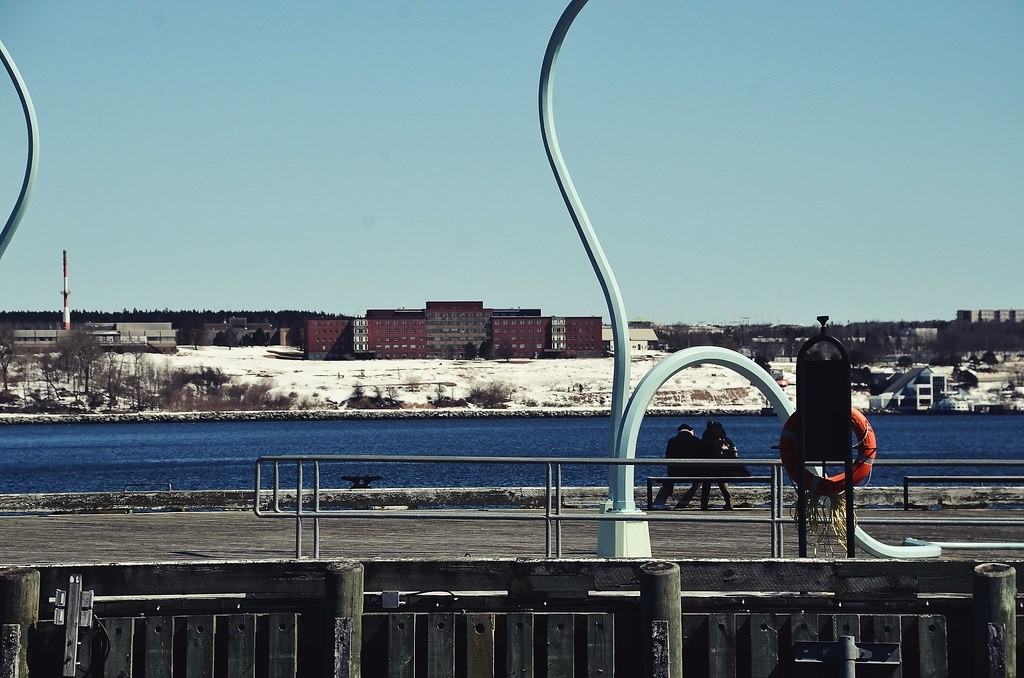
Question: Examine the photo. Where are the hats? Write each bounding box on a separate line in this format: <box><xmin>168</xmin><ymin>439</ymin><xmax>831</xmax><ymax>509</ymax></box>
<box><xmin>678</xmin><ymin>423</ymin><xmax>690</xmax><ymax>429</ymax></box>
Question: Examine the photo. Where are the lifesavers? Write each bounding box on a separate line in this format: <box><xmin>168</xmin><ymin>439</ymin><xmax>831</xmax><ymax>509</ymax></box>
<box><xmin>779</xmin><ymin>406</ymin><xmax>877</xmax><ymax>495</ymax></box>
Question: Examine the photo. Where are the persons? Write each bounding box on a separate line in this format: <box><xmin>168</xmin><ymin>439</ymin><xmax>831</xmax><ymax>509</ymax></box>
<box><xmin>653</xmin><ymin>423</ymin><xmax>702</xmax><ymax>509</ymax></box>
<box><xmin>700</xmin><ymin>421</ymin><xmax>738</xmax><ymax>510</ymax></box>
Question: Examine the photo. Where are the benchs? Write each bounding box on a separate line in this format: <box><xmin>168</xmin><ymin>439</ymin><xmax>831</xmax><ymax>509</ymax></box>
<box><xmin>904</xmin><ymin>476</ymin><xmax>1024</xmax><ymax>510</ymax></box>
<box><xmin>647</xmin><ymin>476</ymin><xmax>772</xmax><ymax>510</ymax></box>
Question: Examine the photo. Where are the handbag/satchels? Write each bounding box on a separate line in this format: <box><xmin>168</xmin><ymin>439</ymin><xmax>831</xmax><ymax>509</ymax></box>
<box><xmin>719</xmin><ymin>434</ymin><xmax>739</xmax><ymax>458</ymax></box>
